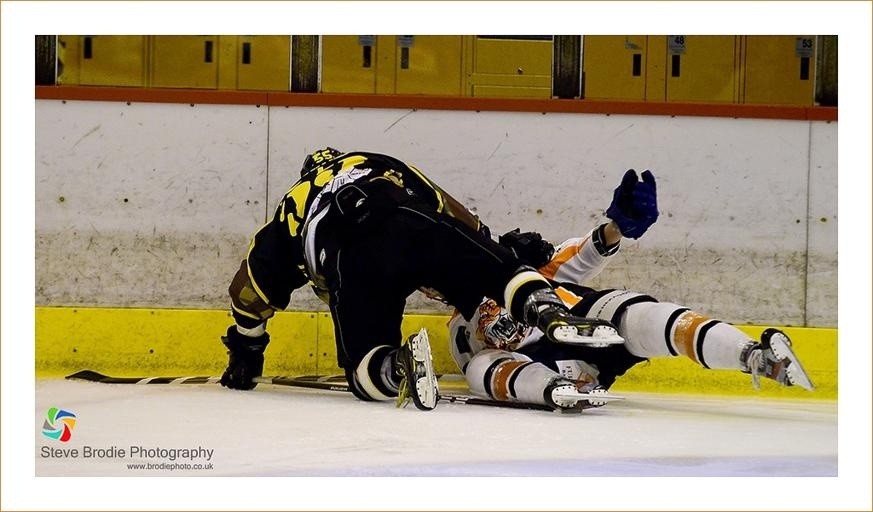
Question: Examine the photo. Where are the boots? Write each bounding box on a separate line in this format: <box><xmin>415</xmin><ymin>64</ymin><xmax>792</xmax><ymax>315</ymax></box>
<box><xmin>738</xmin><ymin>330</ymin><xmax>796</xmax><ymax>385</ymax></box>
<box><xmin>527</xmin><ymin>301</ymin><xmax>621</xmax><ymax>347</ymax></box>
<box><xmin>400</xmin><ymin>334</ymin><xmax>439</xmax><ymax>410</ymax></box>
<box><xmin>543</xmin><ymin>378</ymin><xmax>609</xmax><ymax>410</ymax></box>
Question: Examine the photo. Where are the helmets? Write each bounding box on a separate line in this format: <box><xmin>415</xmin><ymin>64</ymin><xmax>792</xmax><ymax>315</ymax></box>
<box><xmin>300</xmin><ymin>147</ymin><xmax>343</xmax><ymax>177</ymax></box>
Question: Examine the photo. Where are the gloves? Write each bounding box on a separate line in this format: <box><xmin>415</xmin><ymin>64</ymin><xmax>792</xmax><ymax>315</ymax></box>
<box><xmin>498</xmin><ymin>228</ymin><xmax>554</xmax><ymax>266</ymax></box>
<box><xmin>220</xmin><ymin>325</ymin><xmax>270</xmax><ymax>390</ymax></box>
<box><xmin>606</xmin><ymin>169</ymin><xmax>659</xmax><ymax>240</ymax></box>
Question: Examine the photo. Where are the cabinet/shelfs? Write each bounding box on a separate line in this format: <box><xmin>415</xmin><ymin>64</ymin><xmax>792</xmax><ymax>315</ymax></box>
<box><xmin>35</xmin><ymin>34</ymin><xmax>837</xmax><ymax>122</ymax></box>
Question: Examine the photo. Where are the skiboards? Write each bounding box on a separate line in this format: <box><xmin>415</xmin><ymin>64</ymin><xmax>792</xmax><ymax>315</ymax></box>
<box><xmin>249</xmin><ymin>374</ymin><xmax>584</xmax><ymax>415</ymax></box>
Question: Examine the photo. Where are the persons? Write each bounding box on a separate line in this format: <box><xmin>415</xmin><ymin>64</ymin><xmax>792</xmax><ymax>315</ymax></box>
<box><xmin>219</xmin><ymin>146</ymin><xmax>815</xmax><ymax>415</ymax></box>
